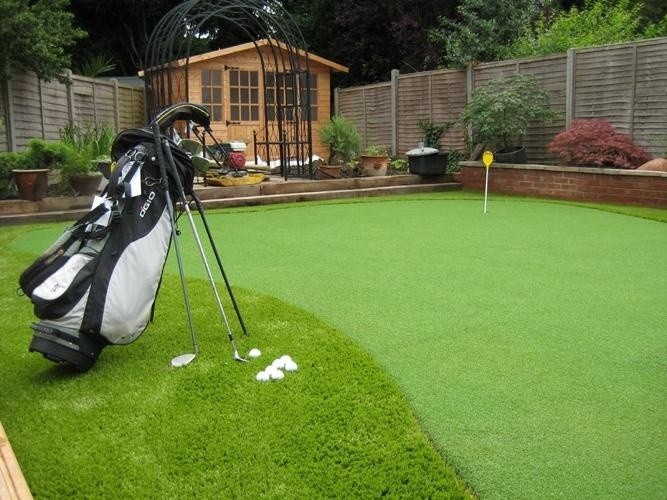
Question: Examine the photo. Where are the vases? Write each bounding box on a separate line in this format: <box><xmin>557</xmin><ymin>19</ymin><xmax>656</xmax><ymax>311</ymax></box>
<box><xmin>98</xmin><ymin>161</ymin><xmax>113</xmax><ymax>179</ymax></box>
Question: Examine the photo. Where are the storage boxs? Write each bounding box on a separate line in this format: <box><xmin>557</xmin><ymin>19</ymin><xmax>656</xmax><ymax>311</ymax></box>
<box><xmin>407</xmin><ymin>152</ymin><xmax>448</xmax><ymax>176</ymax></box>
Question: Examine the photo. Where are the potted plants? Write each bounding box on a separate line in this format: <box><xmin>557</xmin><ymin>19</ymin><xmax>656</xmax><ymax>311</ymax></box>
<box><xmin>48</xmin><ymin>142</ymin><xmax>102</xmax><ymax>196</ymax></box>
<box><xmin>0</xmin><ymin>139</ymin><xmax>58</xmax><ymax>201</ymax></box>
<box><xmin>361</xmin><ymin>144</ymin><xmax>393</xmax><ymax>177</ymax></box>
<box><xmin>458</xmin><ymin>74</ymin><xmax>556</xmax><ymax>162</ymax></box>
<box><xmin>314</xmin><ymin>115</ymin><xmax>361</xmax><ymax>180</ymax></box>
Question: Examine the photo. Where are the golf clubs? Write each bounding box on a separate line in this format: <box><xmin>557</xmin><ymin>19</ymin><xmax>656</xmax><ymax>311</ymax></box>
<box><xmin>162</xmin><ymin>139</ymin><xmax>250</xmax><ymax>363</ymax></box>
<box><xmin>152</xmin><ymin>123</ymin><xmax>198</xmax><ymax>367</ymax></box>
<box><xmin>178</xmin><ymin>139</ymin><xmax>210</xmax><ymax>172</ymax></box>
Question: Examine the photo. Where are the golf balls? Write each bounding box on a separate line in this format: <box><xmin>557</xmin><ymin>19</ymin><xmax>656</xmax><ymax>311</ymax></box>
<box><xmin>249</xmin><ymin>348</ymin><xmax>260</xmax><ymax>357</ymax></box>
<box><xmin>256</xmin><ymin>354</ymin><xmax>297</xmax><ymax>381</ymax></box>
